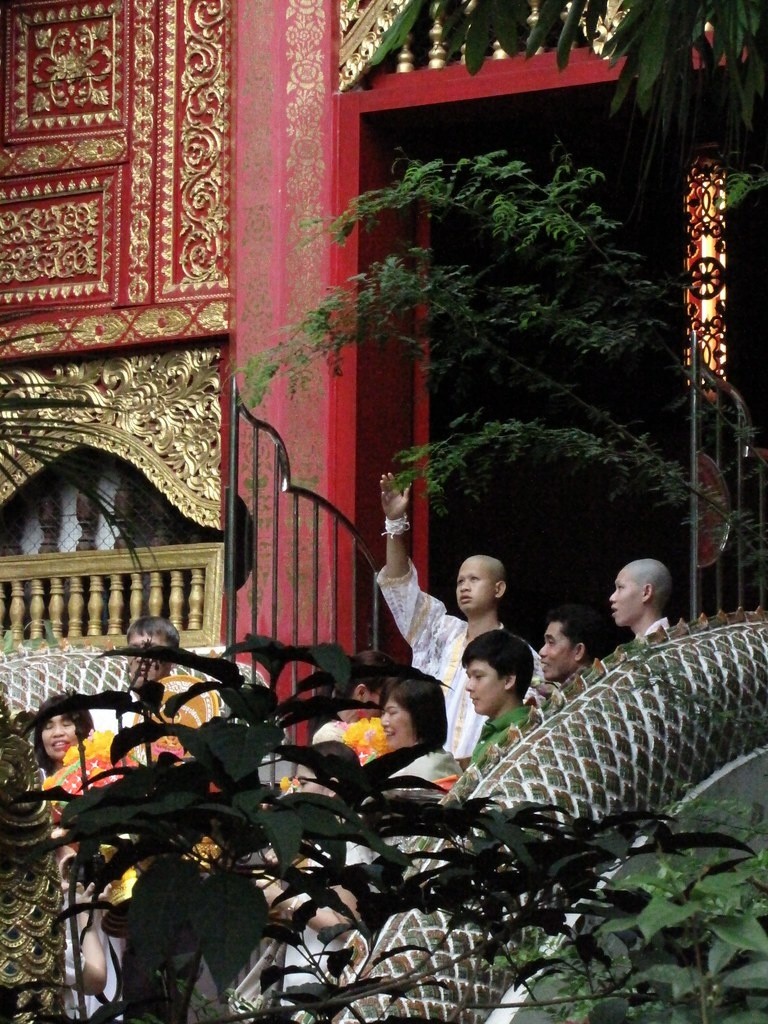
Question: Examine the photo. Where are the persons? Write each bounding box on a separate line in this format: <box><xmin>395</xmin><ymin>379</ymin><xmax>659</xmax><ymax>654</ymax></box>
<box><xmin>36</xmin><ymin>616</ymin><xmax>446</xmax><ymax>1023</ymax></box>
<box><xmin>374</xmin><ymin>468</ymin><xmax>549</xmax><ymax>759</ymax></box>
<box><xmin>608</xmin><ymin>557</ymin><xmax>675</xmax><ymax>643</ymax></box>
<box><xmin>461</xmin><ymin>629</ymin><xmax>535</xmax><ymax>764</ymax></box>
<box><xmin>537</xmin><ymin>607</ymin><xmax>598</xmax><ymax>687</ymax></box>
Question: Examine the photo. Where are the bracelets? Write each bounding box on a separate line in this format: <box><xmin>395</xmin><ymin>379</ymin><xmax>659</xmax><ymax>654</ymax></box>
<box><xmin>382</xmin><ymin>511</ymin><xmax>411</xmax><ymax>538</ymax></box>
